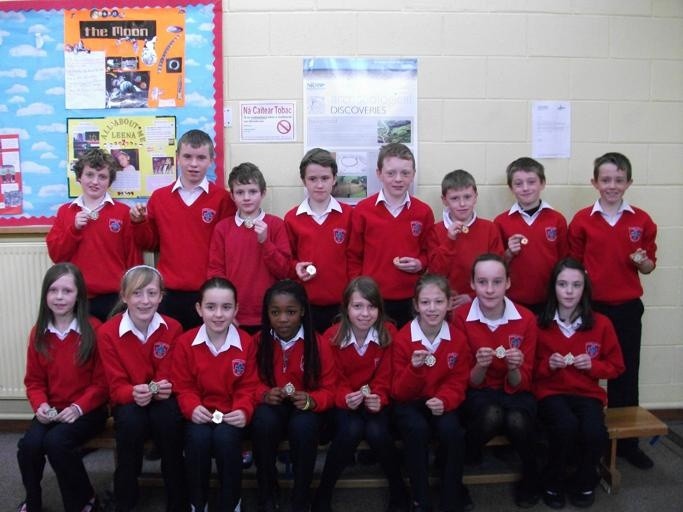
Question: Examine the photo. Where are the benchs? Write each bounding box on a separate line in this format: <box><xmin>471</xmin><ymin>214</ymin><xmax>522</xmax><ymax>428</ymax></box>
<box><xmin>79</xmin><ymin>403</ymin><xmax>669</xmax><ymax>510</ymax></box>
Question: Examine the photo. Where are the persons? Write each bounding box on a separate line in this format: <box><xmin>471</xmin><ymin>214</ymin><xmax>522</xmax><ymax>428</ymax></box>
<box><xmin>14</xmin><ymin>130</ymin><xmax>656</xmax><ymax>508</ymax></box>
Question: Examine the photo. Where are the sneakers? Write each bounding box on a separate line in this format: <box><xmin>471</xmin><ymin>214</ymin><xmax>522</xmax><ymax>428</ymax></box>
<box><xmin>617</xmin><ymin>446</ymin><xmax>654</xmax><ymax>469</ymax></box>
<box><xmin>571</xmin><ymin>484</ymin><xmax>594</xmax><ymax>507</ymax></box>
<box><xmin>517</xmin><ymin>477</ymin><xmax>542</xmax><ymax>509</ymax></box>
<box><xmin>543</xmin><ymin>481</ymin><xmax>565</xmax><ymax>508</ymax></box>
